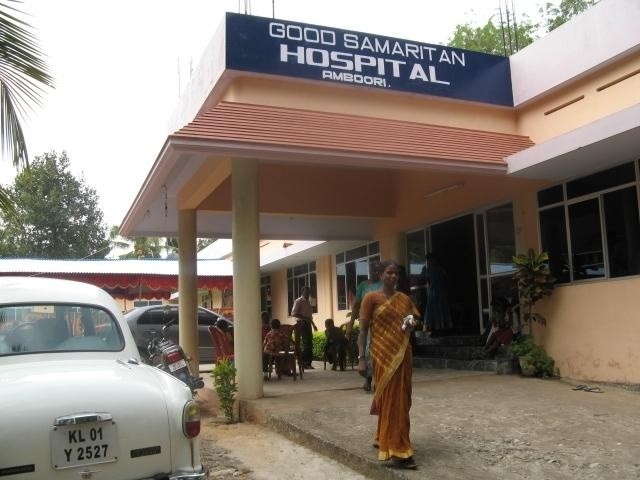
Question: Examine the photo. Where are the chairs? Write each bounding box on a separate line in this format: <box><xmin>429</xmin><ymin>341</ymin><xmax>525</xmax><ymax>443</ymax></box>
<box><xmin>264</xmin><ymin>322</ymin><xmax>306</xmax><ymax>382</ymax></box>
<box><xmin>207</xmin><ymin>323</ymin><xmax>244</xmax><ymax>375</ymax></box>
<box><xmin>325</xmin><ymin>323</ymin><xmax>356</xmax><ymax>370</ymax></box>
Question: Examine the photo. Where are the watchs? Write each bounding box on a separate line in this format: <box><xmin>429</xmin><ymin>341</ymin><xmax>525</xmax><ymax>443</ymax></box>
<box><xmin>357</xmin><ymin>353</ymin><xmax>365</xmax><ymax>360</ymax></box>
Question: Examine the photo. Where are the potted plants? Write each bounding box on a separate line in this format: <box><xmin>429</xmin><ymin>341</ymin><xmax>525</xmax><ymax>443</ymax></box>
<box><xmin>508</xmin><ymin>246</ymin><xmax>553</xmax><ymax>376</ymax></box>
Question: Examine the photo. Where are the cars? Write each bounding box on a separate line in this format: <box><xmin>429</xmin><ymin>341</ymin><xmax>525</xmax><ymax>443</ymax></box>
<box><xmin>96</xmin><ymin>303</ymin><xmax>235</xmax><ymax>362</ymax></box>
<box><xmin>0</xmin><ymin>277</ymin><xmax>204</xmax><ymax>480</ymax></box>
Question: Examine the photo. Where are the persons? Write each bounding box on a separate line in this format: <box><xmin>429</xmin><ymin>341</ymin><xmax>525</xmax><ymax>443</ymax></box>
<box><xmin>475</xmin><ymin>293</ymin><xmax>514</xmax><ymax>359</ymax></box>
<box><xmin>355</xmin><ymin>263</ymin><xmax>425</xmax><ymax>469</ymax></box>
<box><xmin>346</xmin><ymin>261</ymin><xmax>401</xmax><ymax>394</ymax></box>
<box><xmin>216</xmin><ymin>285</ymin><xmax>349</xmax><ymax>372</ymax></box>
<box><xmin>399</xmin><ymin>254</ymin><xmax>452</xmax><ymax>332</ymax></box>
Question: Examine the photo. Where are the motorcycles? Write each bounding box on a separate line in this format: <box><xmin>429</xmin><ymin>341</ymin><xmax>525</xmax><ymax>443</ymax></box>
<box><xmin>143</xmin><ymin>318</ymin><xmax>203</xmax><ymax>397</ymax></box>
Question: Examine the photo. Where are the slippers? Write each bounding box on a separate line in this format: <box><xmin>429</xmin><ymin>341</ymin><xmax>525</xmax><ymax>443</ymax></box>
<box><xmin>571</xmin><ymin>384</ymin><xmax>604</xmax><ymax>393</ymax></box>
